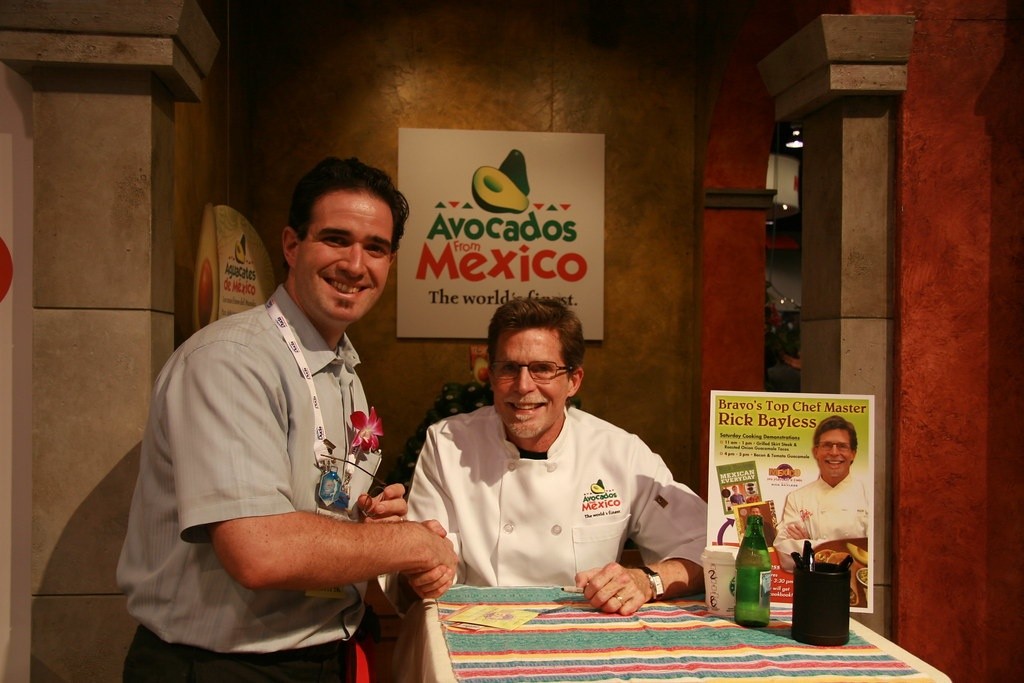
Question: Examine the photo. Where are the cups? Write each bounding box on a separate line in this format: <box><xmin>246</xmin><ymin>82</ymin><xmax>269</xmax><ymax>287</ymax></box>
<box><xmin>700</xmin><ymin>546</ymin><xmax>740</xmax><ymax>616</ymax></box>
<box><xmin>792</xmin><ymin>563</ymin><xmax>852</xmax><ymax>646</ymax></box>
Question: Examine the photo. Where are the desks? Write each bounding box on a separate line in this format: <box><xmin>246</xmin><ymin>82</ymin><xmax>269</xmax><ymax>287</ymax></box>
<box><xmin>393</xmin><ymin>583</ymin><xmax>951</xmax><ymax>683</ymax></box>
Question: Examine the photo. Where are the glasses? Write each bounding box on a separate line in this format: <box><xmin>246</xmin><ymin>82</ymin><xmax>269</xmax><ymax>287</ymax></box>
<box><xmin>492</xmin><ymin>360</ymin><xmax>574</xmax><ymax>381</ymax></box>
<box><xmin>817</xmin><ymin>442</ymin><xmax>854</xmax><ymax>451</ymax></box>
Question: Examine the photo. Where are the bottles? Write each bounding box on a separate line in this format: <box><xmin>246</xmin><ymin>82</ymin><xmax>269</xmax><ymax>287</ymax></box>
<box><xmin>736</xmin><ymin>515</ymin><xmax>772</xmax><ymax>628</ymax></box>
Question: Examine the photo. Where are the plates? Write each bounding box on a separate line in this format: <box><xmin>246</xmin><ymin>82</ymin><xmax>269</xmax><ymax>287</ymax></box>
<box><xmin>856</xmin><ymin>567</ymin><xmax>868</xmax><ymax>587</ymax></box>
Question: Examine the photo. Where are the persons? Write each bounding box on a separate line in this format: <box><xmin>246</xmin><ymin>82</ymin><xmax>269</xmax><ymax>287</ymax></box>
<box><xmin>377</xmin><ymin>297</ymin><xmax>707</xmax><ymax>615</ymax></box>
<box><xmin>773</xmin><ymin>416</ymin><xmax>867</xmax><ymax>574</ymax></box>
<box><xmin>751</xmin><ymin>506</ymin><xmax>773</xmax><ymax>547</ymax></box>
<box><xmin>730</xmin><ymin>485</ymin><xmax>745</xmax><ymax>507</ymax></box>
<box><xmin>116</xmin><ymin>158</ymin><xmax>458</xmax><ymax>683</ymax></box>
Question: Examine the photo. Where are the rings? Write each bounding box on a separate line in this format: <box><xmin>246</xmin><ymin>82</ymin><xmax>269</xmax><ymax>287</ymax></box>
<box><xmin>401</xmin><ymin>517</ymin><xmax>404</xmax><ymax>522</ymax></box>
<box><xmin>614</xmin><ymin>594</ymin><xmax>625</xmax><ymax>606</ymax></box>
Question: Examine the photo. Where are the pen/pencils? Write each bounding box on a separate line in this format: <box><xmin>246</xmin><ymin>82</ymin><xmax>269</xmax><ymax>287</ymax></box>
<box><xmin>561</xmin><ymin>586</ymin><xmax>583</xmax><ymax>593</ymax></box>
<box><xmin>791</xmin><ymin>540</ymin><xmax>855</xmax><ymax>571</ymax></box>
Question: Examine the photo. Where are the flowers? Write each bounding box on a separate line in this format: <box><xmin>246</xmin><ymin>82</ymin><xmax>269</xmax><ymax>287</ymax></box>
<box><xmin>350</xmin><ymin>406</ymin><xmax>384</xmax><ymax>453</ymax></box>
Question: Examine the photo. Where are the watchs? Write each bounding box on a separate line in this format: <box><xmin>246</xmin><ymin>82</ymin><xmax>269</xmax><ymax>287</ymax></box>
<box><xmin>637</xmin><ymin>567</ymin><xmax>664</xmax><ymax>602</ymax></box>
